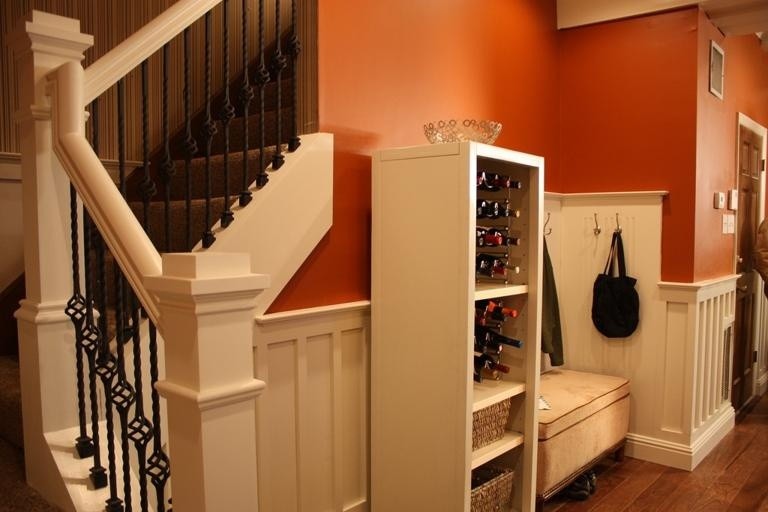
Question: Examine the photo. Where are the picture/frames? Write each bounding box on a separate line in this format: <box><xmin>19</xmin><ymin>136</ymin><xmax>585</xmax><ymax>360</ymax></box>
<box><xmin>709</xmin><ymin>39</ymin><xmax>726</xmax><ymax>99</ymax></box>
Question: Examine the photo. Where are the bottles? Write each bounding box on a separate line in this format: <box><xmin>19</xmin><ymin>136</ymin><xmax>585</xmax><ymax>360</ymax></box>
<box><xmin>476</xmin><ymin>168</ymin><xmax>524</xmax><ymax>279</ymax></box>
<box><xmin>475</xmin><ymin>299</ymin><xmax>523</xmax><ymax>385</ymax></box>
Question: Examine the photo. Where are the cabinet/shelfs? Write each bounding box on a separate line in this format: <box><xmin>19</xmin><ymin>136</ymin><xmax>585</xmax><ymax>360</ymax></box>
<box><xmin>369</xmin><ymin>139</ymin><xmax>548</xmax><ymax>512</ymax></box>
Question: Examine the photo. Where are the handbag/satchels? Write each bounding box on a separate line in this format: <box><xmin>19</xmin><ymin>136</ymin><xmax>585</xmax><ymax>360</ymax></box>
<box><xmin>591</xmin><ymin>274</ymin><xmax>639</xmax><ymax>339</ymax></box>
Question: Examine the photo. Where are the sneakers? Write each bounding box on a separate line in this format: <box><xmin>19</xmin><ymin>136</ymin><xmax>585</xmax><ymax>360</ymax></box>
<box><xmin>565</xmin><ymin>470</ymin><xmax>597</xmax><ymax>500</ymax></box>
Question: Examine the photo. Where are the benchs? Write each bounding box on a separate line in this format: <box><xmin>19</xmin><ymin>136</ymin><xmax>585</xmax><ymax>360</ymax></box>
<box><xmin>538</xmin><ymin>367</ymin><xmax>631</xmax><ymax>502</ymax></box>
